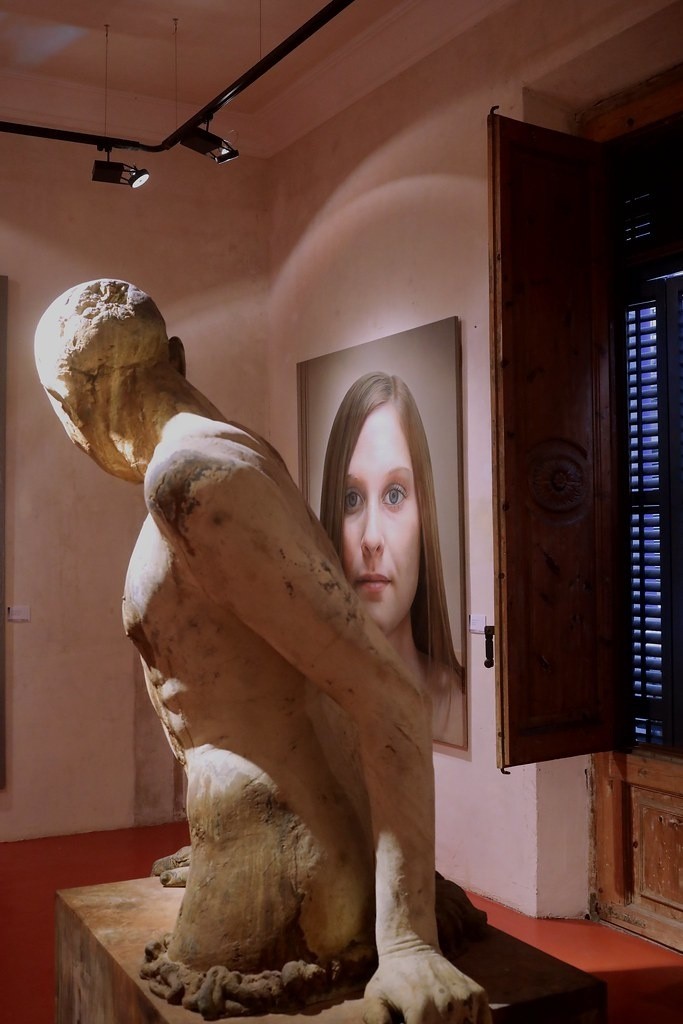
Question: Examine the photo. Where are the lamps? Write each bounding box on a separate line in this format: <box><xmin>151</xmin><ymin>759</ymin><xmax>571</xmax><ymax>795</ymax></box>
<box><xmin>92</xmin><ymin>144</ymin><xmax>150</xmax><ymax>188</ymax></box>
<box><xmin>180</xmin><ymin>114</ymin><xmax>239</xmax><ymax>164</ymax></box>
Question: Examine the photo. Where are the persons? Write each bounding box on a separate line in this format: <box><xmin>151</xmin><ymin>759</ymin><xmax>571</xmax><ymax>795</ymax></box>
<box><xmin>320</xmin><ymin>372</ymin><xmax>464</xmax><ymax>747</ymax></box>
<box><xmin>34</xmin><ymin>279</ymin><xmax>492</xmax><ymax>1024</ymax></box>
